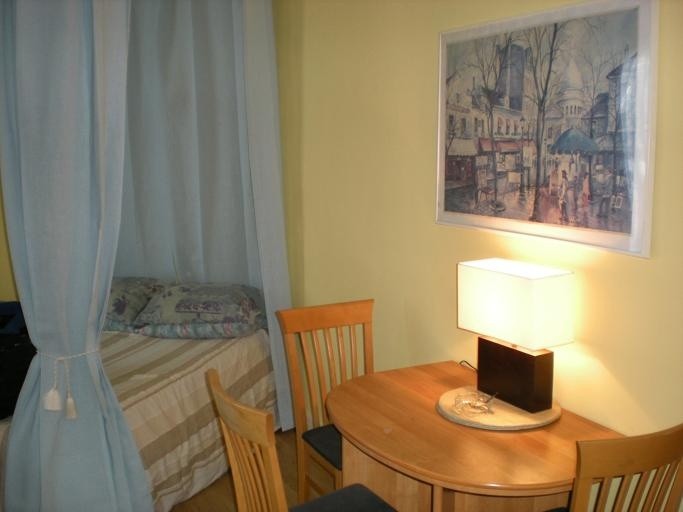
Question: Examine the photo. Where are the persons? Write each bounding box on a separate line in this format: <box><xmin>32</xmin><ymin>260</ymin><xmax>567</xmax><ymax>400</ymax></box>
<box><xmin>570</xmin><ymin>174</ymin><xmax>578</xmax><ymax>213</ymax></box>
<box><xmin>580</xmin><ymin>171</ymin><xmax>591</xmax><ymax>219</ymax></box>
<box><xmin>596</xmin><ymin>167</ymin><xmax>613</xmax><ymax>218</ymax></box>
<box><xmin>556</xmin><ymin>169</ymin><xmax>571</xmax><ymax>223</ymax></box>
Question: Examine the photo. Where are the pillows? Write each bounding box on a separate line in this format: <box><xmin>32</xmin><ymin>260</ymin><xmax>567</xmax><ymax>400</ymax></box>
<box><xmin>131</xmin><ymin>283</ymin><xmax>265</xmax><ymax>340</ymax></box>
<box><xmin>100</xmin><ymin>276</ymin><xmax>163</xmax><ymax>333</ymax></box>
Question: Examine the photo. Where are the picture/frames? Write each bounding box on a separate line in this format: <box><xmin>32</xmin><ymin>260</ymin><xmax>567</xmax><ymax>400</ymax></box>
<box><xmin>433</xmin><ymin>0</ymin><xmax>656</xmax><ymax>258</ymax></box>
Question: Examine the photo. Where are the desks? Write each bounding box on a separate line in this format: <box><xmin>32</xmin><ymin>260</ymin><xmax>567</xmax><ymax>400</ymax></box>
<box><xmin>323</xmin><ymin>359</ymin><xmax>632</xmax><ymax>512</ymax></box>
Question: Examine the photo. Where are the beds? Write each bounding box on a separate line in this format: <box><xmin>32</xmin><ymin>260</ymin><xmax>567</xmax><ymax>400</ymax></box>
<box><xmin>1</xmin><ymin>327</ymin><xmax>280</xmax><ymax>512</ymax></box>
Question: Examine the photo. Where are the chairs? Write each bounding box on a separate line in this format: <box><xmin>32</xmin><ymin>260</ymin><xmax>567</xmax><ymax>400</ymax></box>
<box><xmin>206</xmin><ymin>368</ymin><xmax>399</xmax><ymax>512</ymax></box>
<box><xmin>573</xmin><ymin>425</ymin><xmax>683</xmax><ymax>512</ymax></box>
<box><xmin>273</xmin><ymin>299</ymin><xmax>376</xmax><ymax>500</ymax></box>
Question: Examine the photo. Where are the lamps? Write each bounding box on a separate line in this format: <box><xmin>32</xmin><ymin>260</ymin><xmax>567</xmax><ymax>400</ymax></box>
<box><xmin>435</xmin><ymin>258</ymin><xmax>581</xmax><ymax>431</ymax></box>
